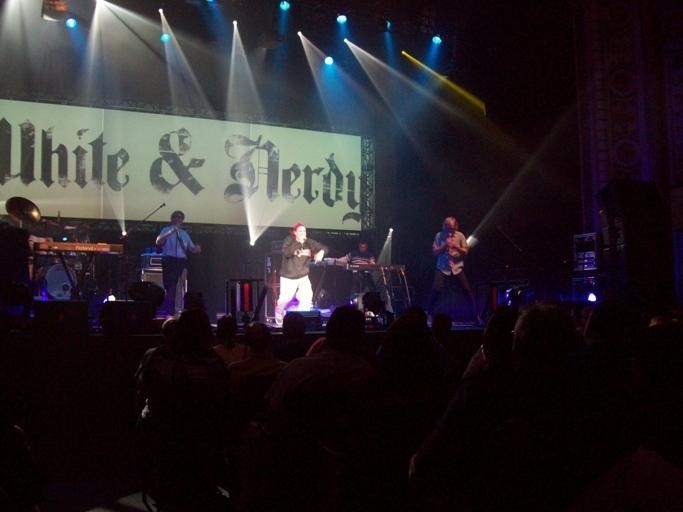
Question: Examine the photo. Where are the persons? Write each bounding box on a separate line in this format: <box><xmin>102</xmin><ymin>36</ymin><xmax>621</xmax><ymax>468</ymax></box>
<box><xmin>155</xmin><ymin>210</ymin><xmax>200</xmax><ymax>321</ymax></box>
<box><xmin>427</xmin><ymin>215</ymin><xmax>486</xmax><ymax>327</ymax></box>
<box><xmin>275</xmin><ymin>224</ymin><xmax>328</xmax><ymax>328</ymax></box>
<box><xmin>339</xmin><ymin>241</ymin><xmax>375</xmax><ymax>306</ymax></box>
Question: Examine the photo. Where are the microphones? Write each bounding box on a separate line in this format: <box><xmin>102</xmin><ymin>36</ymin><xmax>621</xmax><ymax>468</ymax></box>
<box><xmin>448</xmin><ymin>232</ymin><xmax>452</xmax><ymax>244</ymax></box>
<box><xmin>160</xmin><ymin>202</ymin><xmax>166</xmax><ymax>208</ymax></box>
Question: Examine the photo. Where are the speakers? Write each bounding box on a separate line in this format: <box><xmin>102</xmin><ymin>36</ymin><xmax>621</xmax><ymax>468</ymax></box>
<box><xmin>301</xmin><ymin>312</ymin><xmax>321</xmax><ymax>331</ymax></box>
<box><xmin>139</xmin><ymin>268</ymin><xmax>185</xmax><ymax>320</ymax></box>
<box><xmin>34</xmin><ymin>297</ymin><xmax>89</xmax><ymax>330</ymax></box>
<box><xmin>104</xmin><ymin>300</ymin><xmax>152</xmax><ymax>335</ymax></box>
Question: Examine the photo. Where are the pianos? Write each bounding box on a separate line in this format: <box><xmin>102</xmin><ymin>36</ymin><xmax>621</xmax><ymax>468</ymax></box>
<box><xmin>343</xmin><ymin>262</ymin><xmax>406</xmax><ymax>271</ymax></box>
<box><xmin>35</xmin><ymin>241</ymin><xmax>125</xmax><ymax>256</ymax></box>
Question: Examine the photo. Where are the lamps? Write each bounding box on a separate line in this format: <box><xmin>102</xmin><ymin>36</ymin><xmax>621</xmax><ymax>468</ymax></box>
<box><xmin>66</xmin><ymin>10</ymin><xmax>78</xmax><ymax>29</ymax></box>
<box><xmin>432</xmin><ymin>29</ymin><xmax>445</xmax><ymax>45</ymax></box>
<box><xmin>386</xmin><ymin>18</ymin><xmax>392</xmax><ymax>31</ymax></box>
<box><xmin>325</xmin><ymin>56</ymin><xmax>334</xmax><ymax>66</ymax></box>
<box><xmin>280</xmin><ymin>1</ymin><xmax>290</xmax><ymax>11</ymax></box>
<box><xmin>335</xmin><ymin>7</ymin><xmax>347</xmax><ymax>24</ymax></box>
<box><xmin>160</xmin><ymin>27</ymin><xmax>172</xmax><ymax>43</ymax></box>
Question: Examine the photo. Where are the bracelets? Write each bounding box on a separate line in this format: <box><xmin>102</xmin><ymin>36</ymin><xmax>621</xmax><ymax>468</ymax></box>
<box><xmin>169</xmin><ymin>230</ymin><xmax>172</xmax><ymax>234</ymax></box>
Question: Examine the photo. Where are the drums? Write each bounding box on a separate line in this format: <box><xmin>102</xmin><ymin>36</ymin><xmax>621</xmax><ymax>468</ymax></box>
<box><xmin>35</xmin><ymin>264</ymin><xmax>78</xmax><ymax>299</ymax></box>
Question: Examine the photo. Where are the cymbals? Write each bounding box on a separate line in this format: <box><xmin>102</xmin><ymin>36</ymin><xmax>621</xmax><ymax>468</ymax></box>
<box><xmin>6</xmin><ymin>198</ymin><xmax>40</xmax><ymax>226</ymax></box>
